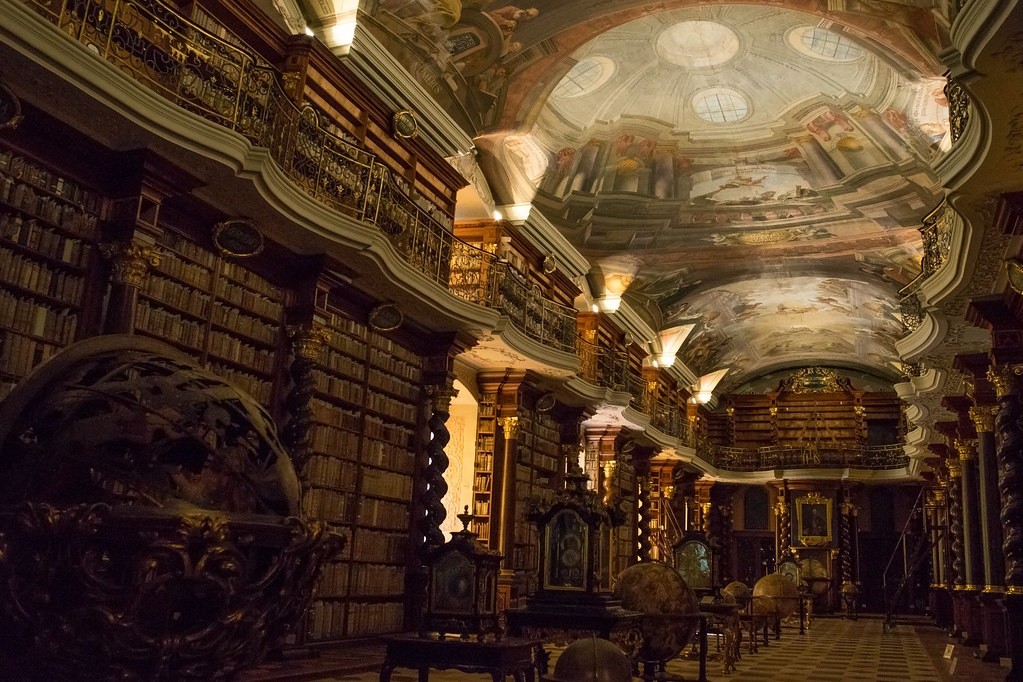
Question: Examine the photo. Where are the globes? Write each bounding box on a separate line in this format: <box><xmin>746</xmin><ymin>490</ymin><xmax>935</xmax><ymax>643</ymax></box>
<box><xmin>554</xmin><ymin>637</ymin><xmax>632</xmax><ymax>682</ymax></box>
<box><xmin>611</xmin><ymin>561</ymin><xmax>700</xmax><ymax>664</ymax></box>
<box><xmin>4</xmin><ymin>333</ymin><xmax>341</xmax><ymax>681</ymax></box>
<box><xmin>724</xmin><ymin>582</ymin><xmax>751</xmax><ymax>610</ymax></box>
<box><xmin>752</xmin><ymin>574</ymin><xmax>799</xmax><ymax>628</ymax></box>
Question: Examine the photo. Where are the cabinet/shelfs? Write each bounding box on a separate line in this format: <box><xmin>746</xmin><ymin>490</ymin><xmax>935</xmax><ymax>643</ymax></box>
<box><xmin>2</xmin><ymin>1</ymin><xmax>1022</xmax><ymax>679</ymax></box>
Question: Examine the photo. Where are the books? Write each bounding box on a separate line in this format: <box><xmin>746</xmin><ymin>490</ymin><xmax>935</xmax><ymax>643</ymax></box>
<box><xmin>492</xmin><ymin>247</ymin><xmax>577</xmax><ymax>356</ymax></box>
<box><xmin>382</xmin><ymin>165</ymin><xmax>484</xmax><ymax>304</ymax></box>
<box><xmin>283</xmin><ymin>95</ymin><xmax>378</xmax><ymax>209</ymax></box>
<box><xmin>1</xmin><ymin>140</ymin><xmax>123</xmax><ymax>405</ymax></box>
<box><xmin>471</xmin><ymin>401</ymin><xmax>581</xmax><ymax>597</ymax></box>
<box><xmin>596</xmin><ymin>460</ymin><xmax>695</xmax><ymax>594</ymax></box>
<box><xmin>304</xmin><ymin>307</ymin><xmax>427</xmax><ymax>639</ymax></box>
<box><xmin>592</xmin><ymin>333</ymin><xmax>688</xmax><ymax>440</ymax></box>
<box><xmin>135</xmin><ymin>222</ymin><xmax>300</xmax><ymax>435</ymax></box>
<box><xmin>179</xmin><ymin>4</ymin><xmax>279</xmax><ymax>148</ymax></box>
